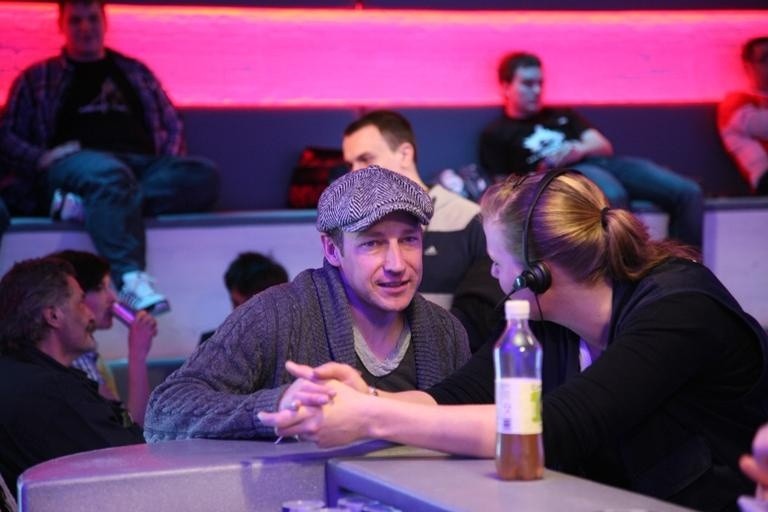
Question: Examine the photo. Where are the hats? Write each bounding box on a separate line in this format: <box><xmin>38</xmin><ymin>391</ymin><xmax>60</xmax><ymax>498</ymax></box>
<box><xmin>314</xmin><ymin>165</ymin><xmax>440</xmax><ymax>236</ymax></box>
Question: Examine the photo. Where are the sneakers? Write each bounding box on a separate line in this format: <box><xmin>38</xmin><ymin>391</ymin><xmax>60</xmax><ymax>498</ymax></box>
<box><xmin>113</xmin><ymin>270</ymin><xmax>173</xmax><ymax>319</ymax></box>
<box><xmin>48</xmin><ymin>188</ymin><xmax>87</xmax><ymax>224</ymax></box>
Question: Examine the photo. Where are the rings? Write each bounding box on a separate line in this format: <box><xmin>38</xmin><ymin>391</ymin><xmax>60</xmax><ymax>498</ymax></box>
<box><xmin>293</xmin><ymin>434</ymin><xmax>302</xmax><ymax>442</ymax></box>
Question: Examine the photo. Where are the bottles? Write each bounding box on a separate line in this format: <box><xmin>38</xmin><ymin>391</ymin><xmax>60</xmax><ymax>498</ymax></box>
<box><xmin>491</xmin><ymin>298</ymin><xmax>546</xmax><ymax>481</ymax></box>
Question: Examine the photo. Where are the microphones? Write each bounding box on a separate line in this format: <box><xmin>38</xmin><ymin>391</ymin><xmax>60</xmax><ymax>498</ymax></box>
<box><xmin>476</xmin><ymin>276</ymin><xmax>524</xmax><ymax>328</ymax></box>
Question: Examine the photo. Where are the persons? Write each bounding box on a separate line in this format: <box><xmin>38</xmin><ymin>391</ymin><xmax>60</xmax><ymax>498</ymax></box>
<box><xmin>0</xmin><ymin>256</ymin><xmax>146</xmax><ymax>512</ymax></box>
<box><xmin>715</xmin><ymin>37</ymin><xmax>768</xmax><ymax>194</ymax></box>
<box><xmin>477</xmin><ymin>52</ymin><xmax>705</xmax><ymax>252</ymax></box>
<box><xmin>142</xmin><ymin>164</ymin><xmax>472</xmax><ymax>445</ymax></box>
<box><xmin>52</xmin><ymin>251</ymin><xmax>157</xmax><ymax>429</ymax></box>
<box><xmin>257</xmin><ymin>168</ymin><xmax>768</xmax><ymax>512</ymax></box>
<box><xmin>735</xmin><ymin>422</ymin><xmax>768</xmax><ymax>512</ymax></box>
<box><xmin>198</xmin><ymin>252</ymin><xmax>288</xmax><ymax>346</ymax></box>
<box><xmin>0</xmin><ymin>0</ymin><xmax>223</xmax><ymax>317</ymax></box>
<box><xmin>341</xmin><ymin>111</ymin><xmax>508</xmax><ymax>353</ymax></box>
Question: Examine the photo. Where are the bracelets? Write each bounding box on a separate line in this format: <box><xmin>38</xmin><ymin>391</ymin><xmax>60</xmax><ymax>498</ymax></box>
<box><xmin>369</xmin><ymin>385</ymin><xmax>378</xmax><ymax>396</ymax></box>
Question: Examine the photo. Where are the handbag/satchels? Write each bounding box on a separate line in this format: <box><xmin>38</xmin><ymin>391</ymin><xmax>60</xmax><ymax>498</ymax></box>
<box><xmin>286</xmin><ymin>145</ymin><xmax>354</xmax><ymax>212</ymax></box>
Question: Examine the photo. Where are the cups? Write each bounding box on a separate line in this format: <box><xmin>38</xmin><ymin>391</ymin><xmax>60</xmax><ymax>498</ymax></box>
<box><xmin>110</xmin><ymin>301</ymin><xmax>137</xmax><ymax>328</ymax></box>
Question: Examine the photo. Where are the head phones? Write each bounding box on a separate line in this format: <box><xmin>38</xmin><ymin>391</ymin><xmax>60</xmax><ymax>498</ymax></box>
<box><xmin>520</xmin><ymin>167</ymin><xmax>589</xmax><ymax>295</ymax></box>
